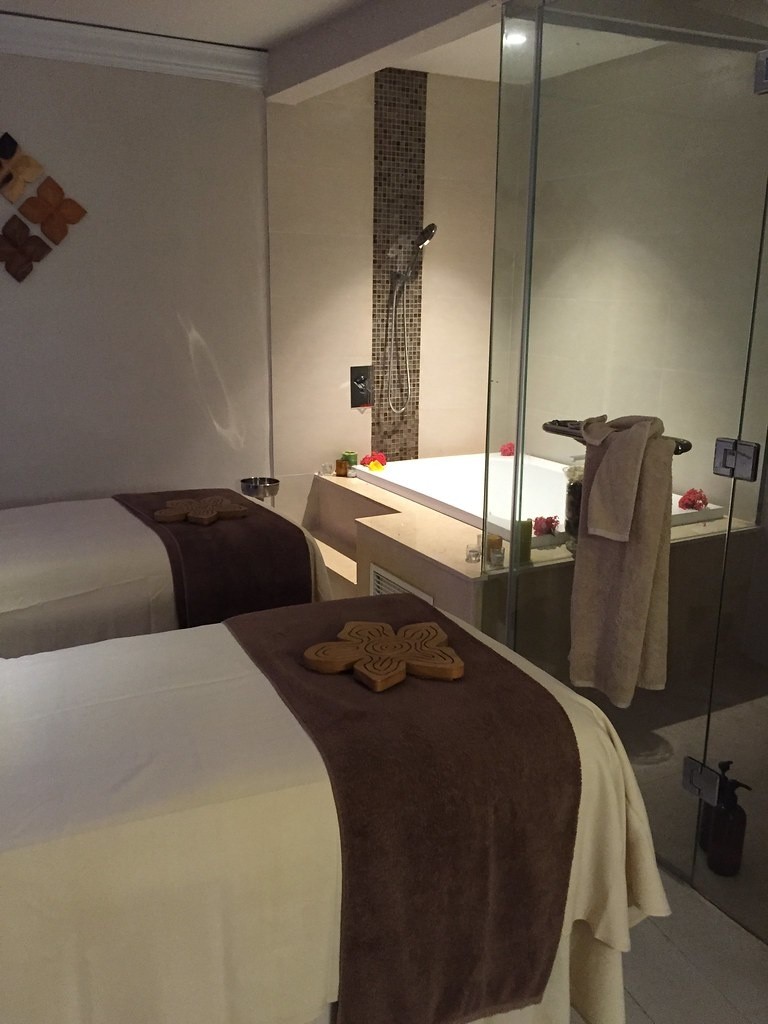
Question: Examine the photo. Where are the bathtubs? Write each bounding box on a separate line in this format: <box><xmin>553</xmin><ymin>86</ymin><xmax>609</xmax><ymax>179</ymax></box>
<box><xmin>349</xmin><ymin>448</ymin><xmax>725</xmax><ymax>548</ymax></box>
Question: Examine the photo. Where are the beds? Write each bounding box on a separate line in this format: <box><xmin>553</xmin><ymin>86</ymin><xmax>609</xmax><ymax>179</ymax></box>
<box><xmin>0</xmin><ymin>485</ymin><xmax>334</xmax><ymax>657</ymax></box>
<box><xmin>0</xmin><ymin>590</ymin><xmax>671</xmax><ymax>1022</ymax></box>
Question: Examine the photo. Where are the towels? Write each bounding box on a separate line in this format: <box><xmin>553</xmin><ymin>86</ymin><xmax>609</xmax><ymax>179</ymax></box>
<box><xmin>564</xmin><ymin>414</ymin><xmax>677</xmax><ymax>710</ymax></box>
<box><xmin>225</xmin><ymin>591</ymin><xmax>584</xmax><ymax>1024</ymax></box>
<box><xmin>111</xmin><ymin>487</ymin><xmax>314</xmax><ymax>628</ymax></box>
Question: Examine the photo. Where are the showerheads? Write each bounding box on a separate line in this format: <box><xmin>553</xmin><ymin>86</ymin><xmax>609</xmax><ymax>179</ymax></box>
<box><xmin>395</xmin><ymin>222</ymin><xmax>438</xmax><ymax>289</ymax></box>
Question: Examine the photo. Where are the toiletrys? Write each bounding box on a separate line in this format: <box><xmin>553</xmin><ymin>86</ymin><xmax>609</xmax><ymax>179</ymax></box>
<box><xmin>695</xmin><ymin>760</ymin><xmax>753</xmax><ymax>878</ymax></box>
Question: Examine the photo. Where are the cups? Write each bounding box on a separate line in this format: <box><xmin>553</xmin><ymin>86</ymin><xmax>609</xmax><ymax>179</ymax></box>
<box><xmin>322</xmin><ymin>464</ymin><xmax>333</xmax><ymax>476</ymax></box>
<box><xmin>342</xmin><ymin>451</ymin><xmax>357</xmax><ymax>466</ymax></box>
<box><xmin>348</xmin><ymin>466</ymin><xmax>356</xmax><ymax>478</ymax></box>
<box><xmin>464</xmin><ymin>534</ymin><xmax>505</xmax><ymax>569</ymax></box>
<box><xmin>336</xmin><ymin>459</ymin><xmax>348</xmax><ymax>476</ymax></box>
